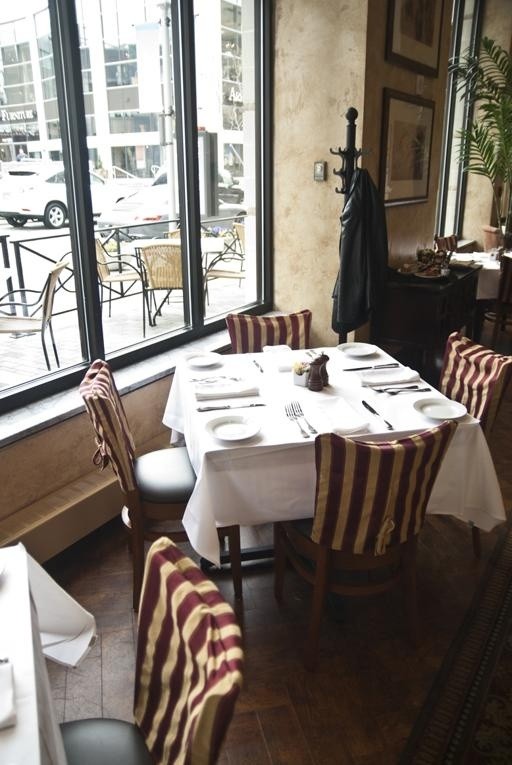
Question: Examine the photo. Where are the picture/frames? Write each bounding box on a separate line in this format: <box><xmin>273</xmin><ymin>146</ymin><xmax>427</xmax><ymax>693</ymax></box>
<box><xmin>384</xmin><ymin>0</ymin><xmax>445</xmax><ymax>80</ymax></box>
<box><xmin>378</xmin><ymin>87</ymin><xmax>437</xmax><ymax>208</ymax></box>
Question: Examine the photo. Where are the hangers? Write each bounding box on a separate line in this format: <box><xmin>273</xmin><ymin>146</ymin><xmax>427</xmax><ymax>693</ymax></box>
<box><xmin>328</xmin><ymin>106</ymin><xmax>373</xmax><ymax>344</ymax></box>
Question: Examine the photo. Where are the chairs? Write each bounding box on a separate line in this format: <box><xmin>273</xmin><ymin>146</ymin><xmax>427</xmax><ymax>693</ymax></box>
<box><xmin>225</xmin><ymin>308</ymin><xmax>312</xmax><ymax>355</ymax></box>
<box><xmin>473</xmin><ymin>255</ymin><xmax>511</xmax><ymax>349</ymax></box>
<box><xmin>94</xmin><ymin>238</ymin><xmax>157</xmax><ymax>325</ymax></box>
<box><xmin>204</xmin><ymin>222</ymin><xmax>245</xmax><ymax>307</ymax></box>
<box><xmin>436</xmin><ymin>234</ymin><xmax>457</xmax><ymax>255</ymax></box>
<box><xmin>482</xmin><ymin>225</ymin><xmax>501</xmax><ymax>251</ymax></box>
<box><xmin>137</xmin><ymin>243</ymin><xmax>181</xmax><ymax>339</ymax></box>
<box><xmin>272</xmin><ymin>419</ymin><xmax>457</xmax><ymax>674</ymax></box>
<box><xmin>57</xmin><ymin>535</ymin><xmax>244</xmax><ymax>764</ymax></box>
<box><xmin>0</xmin><ymin>261</ymin><xmax>70</xmax><ymax>372</ymax></box>
<box><xmin>438</xmin><ymin>330</ymin><xmax>512</xmax><ymax>562</ymax></box>
<box><xmin>78</xmin><ymin>359</ymin><xmax>199</xmax><ymax>615</ymax></box>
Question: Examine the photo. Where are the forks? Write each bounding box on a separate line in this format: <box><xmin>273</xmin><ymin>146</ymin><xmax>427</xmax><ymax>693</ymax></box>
<box><xmin>386</xmin><ymin>387</ymin><xmax>430</xmax><ymax>395</ymax></box>
<box><xmin>284</xmin><ymin>404</ymin><xmax>309</xmax><ymax>438</ymax></box>
<box><xmin>292</xmin><ymin>402</ymin><xmax>317</xmax><ymax>434</ymax></box>
<box><xmin>370</xmin><ymin>385</ymin><xmax>418</xmax><ymax>393</ymax></box>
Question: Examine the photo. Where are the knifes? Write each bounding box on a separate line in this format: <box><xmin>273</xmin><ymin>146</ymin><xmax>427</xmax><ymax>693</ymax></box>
<box><xmin>362</xmin><ymin>400</ymin><xmax>393</xmax><ymax>429</ymax></box>
<box><xmin>196</xmin><ymin>403</ymin><xmax>265</xmax><ymax>412</ymax></box>
<box><xmin>343</xmin><ymin>363</ymin><xmax>399</xmax><ymax>372</ymax></box>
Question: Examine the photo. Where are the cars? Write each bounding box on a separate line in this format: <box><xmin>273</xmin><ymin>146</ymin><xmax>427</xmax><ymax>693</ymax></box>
<box><xmin>97</xmin><ymin>184</ymin><xmax>247</xmax><ymax>242</ymax></box>
<box><xmin>0</xmin><ymin>161</ymin><xmax>137</xmax><ymax>229</ymax></box>
<box><xmin>216</xmin><ymin>164</ymin><xmax>244</xmax><ymax>204</ymax></box>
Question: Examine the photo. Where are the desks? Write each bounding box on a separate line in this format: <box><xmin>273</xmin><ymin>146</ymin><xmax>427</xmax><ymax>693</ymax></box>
<box><xmin>376</xmin><ymin>261</ymin><xmax>483</xmax><ymax>375</ymax></box>
<box><xmin>132</xmin><ymin>237</ymin><xmax>225</xmax><ymax>269</ymax></box>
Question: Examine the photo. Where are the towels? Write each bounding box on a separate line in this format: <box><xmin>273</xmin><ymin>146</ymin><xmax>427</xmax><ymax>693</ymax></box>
<box><xmin>195</xmin><ymin>377</ymin><xmax>259</xmax><ymax>399</ymax></box>
<box><xmin>0</xmin><ymin>664</ymin><xmax>17</xmax><ymax>729</ymax></box>
<box><xmin>263</xmin><ymin>345</ymin><xmax>299</xmax><ymax>370</ymax></box>
<box><xmin>317</xmin><ymin>396</ymin><xmax>370</xmax><ymax>433</ymax></box>
<box><xmin>359</xmin><ymin>367</ymin><xmax>420</xmax><ymax>386</ymax></box>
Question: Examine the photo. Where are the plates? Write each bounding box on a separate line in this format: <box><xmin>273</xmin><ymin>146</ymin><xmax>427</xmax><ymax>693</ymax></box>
<box><xmin>204</xmin><ymin>415</ymin><xmax>261</xmax><ymax>442</ymax></box>
<box><xmin>412</xmin><ymin>398</ymin><xmax>467</xmax><ymax>420</ymax></box>
<box><xmin>336</xmin><ymin>342</ymin><xmax>377</xmax><ymax>358</ymax></box>
<box><xmin>183</xmin><ymin>351</ymin><xmax>222</xmax><ymax>368</ymax></box>
<box><xmin>396</xmin><ymin>264</ymin><xmax>418</xmax><ymax>275</ymax></box>
